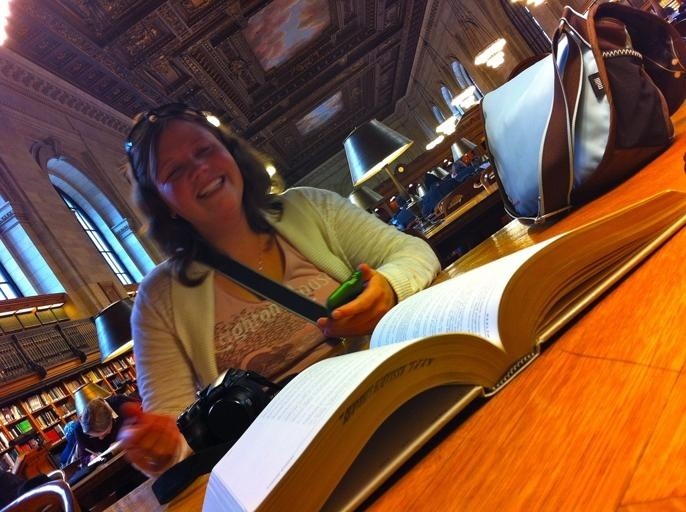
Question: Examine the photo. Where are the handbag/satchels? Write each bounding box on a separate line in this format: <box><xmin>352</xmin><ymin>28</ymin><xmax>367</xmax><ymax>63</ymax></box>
<box><xmin>479</xmin><ymin>1</ymin><xmax>685</xmax><ymax>227</ymax></box>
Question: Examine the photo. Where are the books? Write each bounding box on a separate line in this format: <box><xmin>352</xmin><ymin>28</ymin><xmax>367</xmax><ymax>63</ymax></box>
<box><xmin>198</xmin><ymin>179</ymin><xmax>685</xmax><ymax>512</ymax></box>
<box><xmin>659</xmin><ymin>0</ymin><xmax>686</xmax><ymax>22</ymax></box>
<box><xmin>0</xmin><ymin>351</ymin><xmax>136</xmax><ymax>473</ymax></box>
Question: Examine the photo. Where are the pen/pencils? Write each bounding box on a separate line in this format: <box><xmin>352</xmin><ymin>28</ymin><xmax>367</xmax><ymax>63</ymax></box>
<box><xmin>84</xmin><ymin>447</ymin><xmax>98</xmax><ymax>457</ymax></box>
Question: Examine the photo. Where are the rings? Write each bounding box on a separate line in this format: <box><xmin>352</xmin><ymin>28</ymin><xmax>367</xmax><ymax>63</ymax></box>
<box><xmin>141</xmin><ymin>456</ymin><xmax>157</xmax><ymax>468</ymax></box>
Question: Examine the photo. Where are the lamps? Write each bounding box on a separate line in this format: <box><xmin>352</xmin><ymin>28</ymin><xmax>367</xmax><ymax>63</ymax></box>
<box><xmin>343</xmin><ymin>114</ymin><xmax>482</xmax><ymax>246</ymax></box>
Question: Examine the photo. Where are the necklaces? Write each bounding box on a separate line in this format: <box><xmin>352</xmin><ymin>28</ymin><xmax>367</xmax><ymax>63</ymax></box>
<box><xmin>249</xmin><ymin>236</ymin><xmax>265</xmax><ymax>272</ymax></box>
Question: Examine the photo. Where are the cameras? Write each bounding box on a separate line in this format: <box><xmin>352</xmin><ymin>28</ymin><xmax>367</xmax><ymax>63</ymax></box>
<box><xmin>176</xmin><ymin>368</ymin><xmax>282</xmax><ymax>468</ymax></box>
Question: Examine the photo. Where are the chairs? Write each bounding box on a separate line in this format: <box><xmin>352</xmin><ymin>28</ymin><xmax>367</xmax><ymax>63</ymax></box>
<box><xmin>0</xmin><ymin>477</ymin><xmax>82</xmax><ymax>512</ymax></box>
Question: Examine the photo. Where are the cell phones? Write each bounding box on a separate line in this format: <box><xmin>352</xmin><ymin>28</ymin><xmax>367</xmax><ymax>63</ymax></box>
<box><xmin>327</xmin><ymin>271</ymin><xmax>362</xmax><ymax>319</ymax></box>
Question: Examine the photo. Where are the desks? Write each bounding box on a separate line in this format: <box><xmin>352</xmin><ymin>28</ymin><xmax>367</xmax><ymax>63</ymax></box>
<box><xmin>101</xmin><ymin>95</ymin><xmax>685</xmax><ymax>512</ymax></box>
<box><xmin>422</xmin><ymin>183</ymin><xmax>505</xmax><ymax>250</ymax></box>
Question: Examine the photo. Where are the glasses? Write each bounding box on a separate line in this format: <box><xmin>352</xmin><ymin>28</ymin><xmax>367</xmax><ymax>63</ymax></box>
<box><xmin>125</xmin><ymin>102</ymin><xmax>206</xmax><ymax>181</ymax></box>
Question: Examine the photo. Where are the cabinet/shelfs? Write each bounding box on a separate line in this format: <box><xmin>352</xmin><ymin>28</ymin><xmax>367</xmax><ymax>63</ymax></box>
<box><xmin>0</xmin><ymin>281</ymin><xmax>142</xmax><ymax>474</ymax></box>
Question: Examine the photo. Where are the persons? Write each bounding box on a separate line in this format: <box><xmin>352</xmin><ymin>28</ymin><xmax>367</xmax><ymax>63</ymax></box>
<box><xmin>118</xmin><ymin>104</ymin><xmax>442</xmax><ymax>479</ymax></box>
<box><xmin>390</xmin><ymin>160</ymin><xmax>475</xmax><ymax>230</ymax></box>
